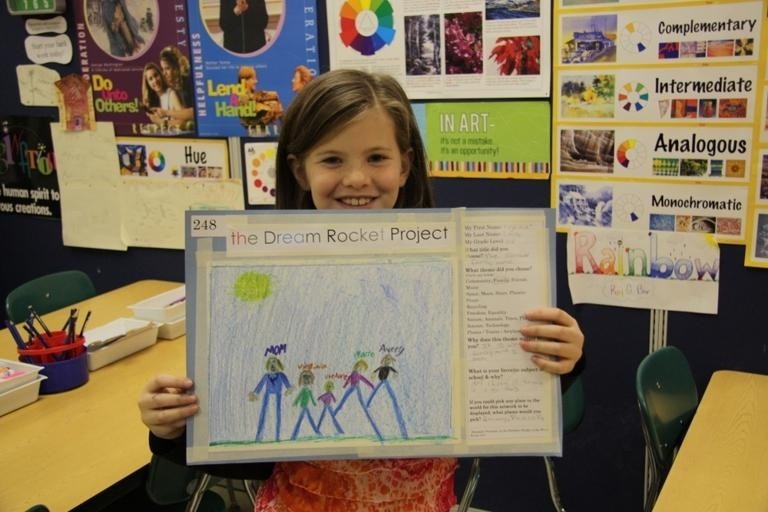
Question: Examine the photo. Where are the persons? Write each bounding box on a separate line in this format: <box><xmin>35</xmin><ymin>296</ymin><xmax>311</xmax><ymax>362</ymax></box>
<box><xmin>238</xmin><ymin>65</ymin><xmax>285</xmax><ymax>130</ymax></box>
<box><xmin>101</xmin><ymin>0</ymin><xmax>196</xmax><ymax>131</ymax></box>
<box><xmin>220</xmin><ymin>0</ymin><xmax>268</xmax><ymax>54</ymax></box>
<box><xmin>137</xmin><ymin>68</ymin><xmax>583</xmax><ymax>512</ymax></box>
<box><xmin>291</xmin><ymin>65</ymin><xmax>314</xmax><ymax>94</ymax></box>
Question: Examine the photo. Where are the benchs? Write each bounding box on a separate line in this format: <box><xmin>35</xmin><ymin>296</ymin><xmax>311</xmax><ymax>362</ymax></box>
<box><xmin>0</xmin><ymin>279</ymin><xmax>190</xmax><ymax>512</ymax></box>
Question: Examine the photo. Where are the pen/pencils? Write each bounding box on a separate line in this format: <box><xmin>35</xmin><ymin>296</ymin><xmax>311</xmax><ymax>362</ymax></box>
<box><xmin>5</xmin><ymin>305</ymin><xmax>92</xmax><ymax>364</ymax></box>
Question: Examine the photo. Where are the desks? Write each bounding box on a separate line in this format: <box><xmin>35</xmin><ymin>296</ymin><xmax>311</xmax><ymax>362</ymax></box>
<box><xmin>651</xmin><ymin>370</ymin><xmax>768</xmax><ymax>512</ymax></box>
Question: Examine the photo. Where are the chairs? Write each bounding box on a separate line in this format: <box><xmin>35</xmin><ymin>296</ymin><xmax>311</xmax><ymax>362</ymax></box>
<box><xmin>632</xmin><ymin>346</ymin><xmax>700</xmax><ymax>512</ymax></box>
<box><xmin>6</xmin><ymin>270</ymin><xmax>96</xmax><ymax>325</ymax></box>
